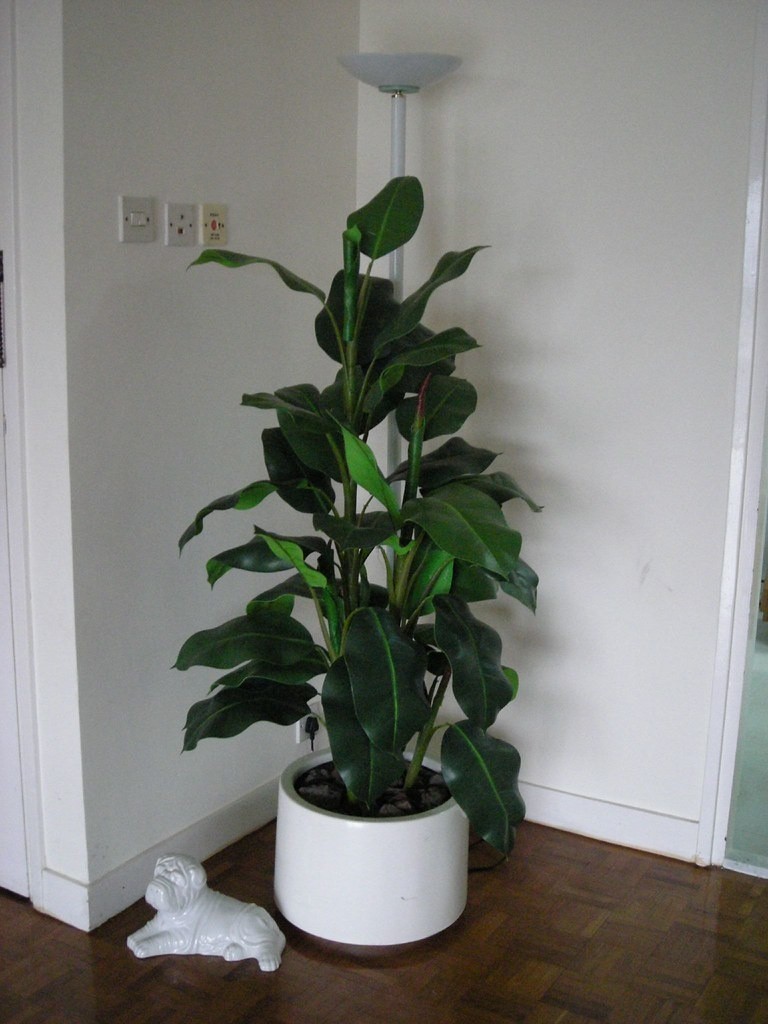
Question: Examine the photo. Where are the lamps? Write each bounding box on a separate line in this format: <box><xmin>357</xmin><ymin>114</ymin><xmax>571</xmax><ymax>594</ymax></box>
<box><xmin>336</xmin><ymin>52</ymin><xmax>462</xmax><ymax>562</ymax></box>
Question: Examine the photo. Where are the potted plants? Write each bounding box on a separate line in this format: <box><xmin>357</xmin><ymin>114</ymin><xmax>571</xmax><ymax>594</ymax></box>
<box><xmin>171</xmin><ymin>175</ymin><xmax>544</xmax><ymax>957</ymax></box>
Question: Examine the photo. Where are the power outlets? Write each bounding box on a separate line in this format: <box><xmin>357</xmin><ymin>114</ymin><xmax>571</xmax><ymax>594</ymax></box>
<box><xmin>296</xmin><ymin>702</ymin><xmax>320</xmax><ymax>745</ymax></box>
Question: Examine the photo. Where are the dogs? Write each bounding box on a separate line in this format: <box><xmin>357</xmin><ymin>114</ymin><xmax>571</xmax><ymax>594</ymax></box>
<box><xmin>127</xmin><ymin>854</ymin><xmax>285</xmax><ymax>972</ymax></box>
